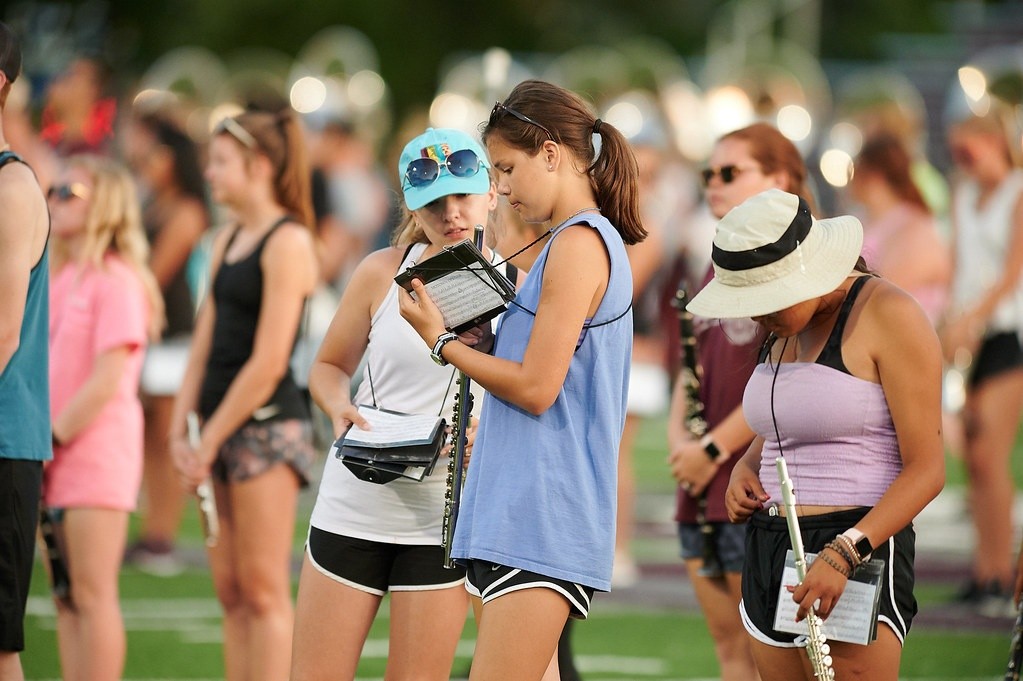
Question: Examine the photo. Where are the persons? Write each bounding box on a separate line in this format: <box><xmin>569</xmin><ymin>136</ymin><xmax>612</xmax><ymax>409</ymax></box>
<box><xmin>1</xmin><ymin>22</ymin><xmax>1023</xmax><ymax>681</ymax></box>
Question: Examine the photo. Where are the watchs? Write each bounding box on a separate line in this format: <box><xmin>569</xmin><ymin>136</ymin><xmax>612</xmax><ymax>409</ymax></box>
<box><xmin>700</xmin><ymin>434</ymin><xmax>731</xmax><ymax>465</ymax></box>
<box><xmin>429</xmin><ymin>332</ymin><xmax>458</xmax><ymax>366</ymax></box>
<box><xmin>843</xmin><ymin>527</ymin><xmax>873</xmax><ymax>562</ymax></box>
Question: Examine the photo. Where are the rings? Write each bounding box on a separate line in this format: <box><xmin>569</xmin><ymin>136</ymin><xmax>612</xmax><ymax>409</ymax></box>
<box><xmin>681</xmin><ymin>481</ymin><xmax>693</xmax><ymax>491</ymax></box>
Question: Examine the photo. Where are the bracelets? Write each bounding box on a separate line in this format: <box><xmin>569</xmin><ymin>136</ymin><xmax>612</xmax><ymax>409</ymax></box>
<box><xmin>818</xmin><ymin>550</ymin><xmax>849</xmax><ymax>576</ymax></box>
<box><xmin>828</xmin><ymin>537</ymin><xmax>856</xmax><ymax>576</ymax></box>
<box><xmin>824</xmin><ymin>543</ymin><xmax>854</xmax><ymax>574</ymax></box>
<box><xmin>836</xmin><ymin>533</ymin><xmax>860</xmax><ymax>568</ymax></box>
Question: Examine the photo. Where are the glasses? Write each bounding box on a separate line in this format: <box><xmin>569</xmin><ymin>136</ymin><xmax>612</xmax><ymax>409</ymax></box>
<box><xmin>214</xmin><ymin>115</ymin><xmax>260</xmax><ymax>151</ymax></box>
<box><xmin>47</xmin><ymin>182</ymin><xmax>86</xmax><ymax>207</ymax></box>
<box><xmin>401</xmin><ymin>148</ymin><xmax>492</xmax><ymax>196</ymax></box>
<box><xmin>488</xmin><ymin>100</ymin><xmax>556</xmax><ymax>145</ymax></box>
<box><xmin>701</xmin><ymin>166</ymin><xmax>745</xmax><ymax>185</ymax></box>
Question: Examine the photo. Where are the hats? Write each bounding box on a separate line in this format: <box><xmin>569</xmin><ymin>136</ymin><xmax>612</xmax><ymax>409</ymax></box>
<box><xmin>396</xmin><ymin>126</ymin><xmax>493</xmax><ymax>210</ymax></box>
<box><xmin>685</xmin><ymin>187</ymin><xmax>866</xmax><ymax>319</ymax></box>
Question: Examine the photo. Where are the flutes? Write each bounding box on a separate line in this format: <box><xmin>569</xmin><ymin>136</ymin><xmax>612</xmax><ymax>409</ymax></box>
<box><xmin>38</xmin><ymin>503</ymin><xmax>71</xmax><ymax>601</ymax></box>
<box><xmin>668</xmin><ymin>279</ymin><xmax>714</xmax><ymax>569</ymax></box>
<box><xmin>439</xmin><ymin>369</ymin><xmax>475</xmax><ymax>572</ymax></box>
<box><xmin>773</xmin><ymin>454</ymin><xmax>835</xmax><ymax>681</ymax></box>
<box><xmin>185</xmin><ymin>409</ymin><xmax>221</xmax><ymax>548</ymax></box>
